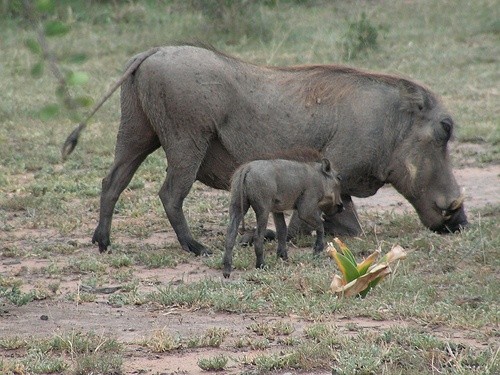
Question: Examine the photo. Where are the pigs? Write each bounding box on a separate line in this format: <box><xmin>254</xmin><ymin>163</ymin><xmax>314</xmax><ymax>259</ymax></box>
<box><xmin>62</xmin><ymin>36</ymin><xmax>467</xmax><ymax>257</ymax></box>
<box><xmin>222</xmin><ymin>146</ymin><xmax>346</xmax><ymax>280</ymax></box>
